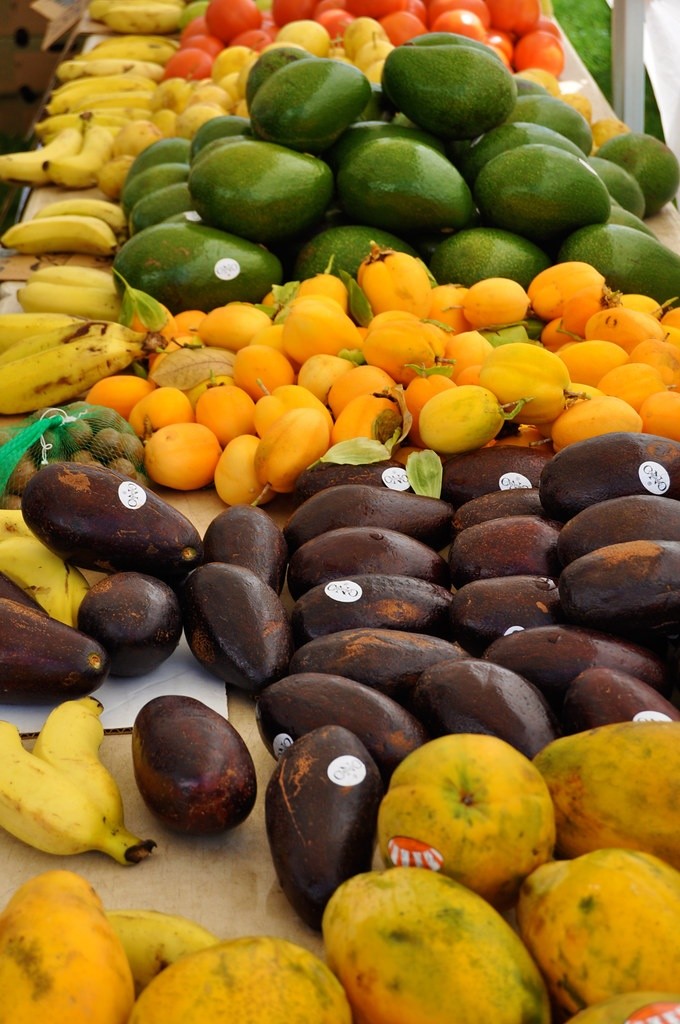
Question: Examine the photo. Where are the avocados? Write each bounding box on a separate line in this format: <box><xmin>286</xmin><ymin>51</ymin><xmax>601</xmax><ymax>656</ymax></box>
<box><xmin>0</xmin><ymin>429</ymin><xmax>679</xmax><ymax>925</ymax></box>
<box><xmin>110</xmin><ymin>29</ymin><xmax>679</xmax><ymax>306</ymax></box>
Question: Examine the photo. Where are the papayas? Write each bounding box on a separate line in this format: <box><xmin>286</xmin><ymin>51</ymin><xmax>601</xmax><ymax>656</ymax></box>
<box><xmin>0</xmin><ymin>720</ymin><xmax>680</xmax><ymax>1024</ymax></box>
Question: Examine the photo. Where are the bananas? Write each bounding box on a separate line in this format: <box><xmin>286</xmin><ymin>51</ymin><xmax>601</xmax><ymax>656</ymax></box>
<box><xmin>0</xmin><ymin>509</ymin><xmax>95</xmax><ymax>631</ymax></box>
<box><xmin>0</xmin><ymin>695</ymin><xmax>160</xmax><ymax>865</ymax></box>
<box><xmin>0</xmin><ymin>0</ymin><xmax>183</xmax><ymax>417</ymax></box>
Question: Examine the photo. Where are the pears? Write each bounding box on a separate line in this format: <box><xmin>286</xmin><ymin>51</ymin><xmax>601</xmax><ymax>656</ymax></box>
<box><xmin>97</xmin><ymin>16</ymin><xmax>634</xmax><ymax>202</ymax></box>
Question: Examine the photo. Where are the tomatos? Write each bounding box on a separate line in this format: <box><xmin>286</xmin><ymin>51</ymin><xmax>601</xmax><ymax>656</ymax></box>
<box><xmin>160</xmin><ymin>0</ymin><xmax>571</xmax><ymax>77</ymax></box>
